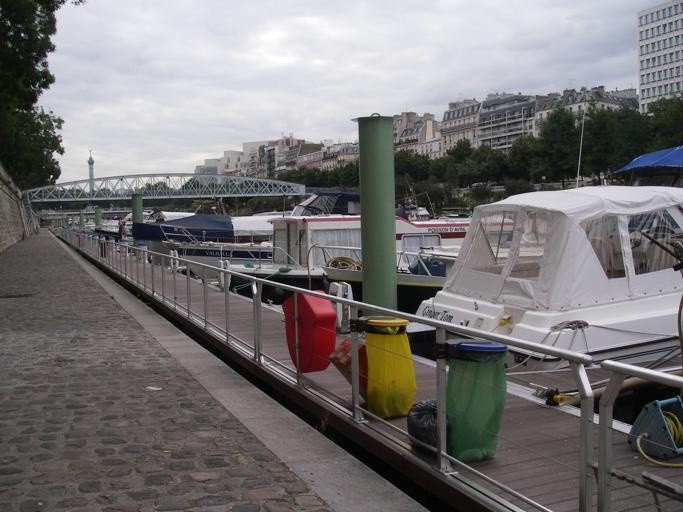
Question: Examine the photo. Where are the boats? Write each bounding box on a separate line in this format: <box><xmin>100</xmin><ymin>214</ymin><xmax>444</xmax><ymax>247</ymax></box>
<box><xmin>216</xmin><ymin>214</ymin><xmax>458</xmax><ymax>310</ymax></box>
<box><xmin>405</xmin><ymin>186</ymin><xmax>683</xmax><ymax>375</ymax></box>
<box><xmin>91</xmin><ymin>190</ymin><xmax>512</xmax><ymax>277</ymax></box>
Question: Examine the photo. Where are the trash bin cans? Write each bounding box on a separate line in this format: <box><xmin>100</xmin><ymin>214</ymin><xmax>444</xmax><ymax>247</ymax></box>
<box><xmin>445</xmin><ymin>338</ymin><xmax>507</xmax><ymax>463</ymax></box>
<box><xmin>363</xmin><ymin>317</ymin><xmax>418</xmax><ymax>419</ymax></box>
